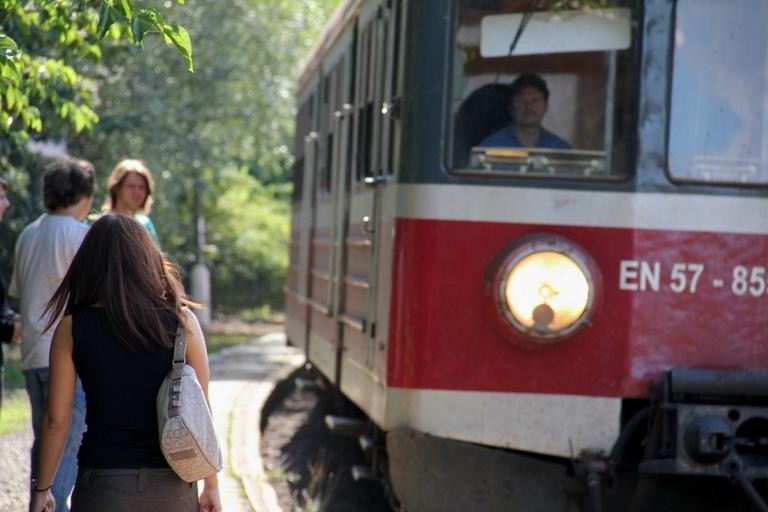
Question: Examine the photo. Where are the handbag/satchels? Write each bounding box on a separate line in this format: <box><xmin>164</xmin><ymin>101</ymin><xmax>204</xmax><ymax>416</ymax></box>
<box><xmin>155</xmin><ymin>305</ymin><xmax>225</xmax><ymax>484</ymax></box>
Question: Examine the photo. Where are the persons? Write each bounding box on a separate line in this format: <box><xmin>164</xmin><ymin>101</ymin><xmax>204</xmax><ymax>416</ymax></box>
<box><xmin>468</xmin><ymin>72</ymin><xmax>583</xmax><ymax>175</ymax></box>
<box><xmin>29</xmin><ymin>213</ymin><xmax>222</xmax><ymax>512</ymax></box>
<box><xmin>0</xmin><ymin>179</ymin><xmax>23</xmax><ymax>408</ymax></box>
<box><xmin>7</xmin><ymin>157</ymin><xmax>97</xmax><ymax>512</ymax></box>
<box><xmin>82</xmin><ymin>159</ymin><xmax>162</xmax><ymax>252</ymax></box>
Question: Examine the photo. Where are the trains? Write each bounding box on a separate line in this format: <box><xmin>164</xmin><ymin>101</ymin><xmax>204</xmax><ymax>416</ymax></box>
<box><xmin>287</xmin><ymin>0</ymin><xmax>768</xmax><ymax>512</ymax></box>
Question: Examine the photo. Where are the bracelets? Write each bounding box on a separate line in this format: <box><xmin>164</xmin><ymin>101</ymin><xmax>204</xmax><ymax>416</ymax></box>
<box><xmin>34</xmin><ymin>483</ymin><xmax>52</xmax><ymax>492</ymax></box>
